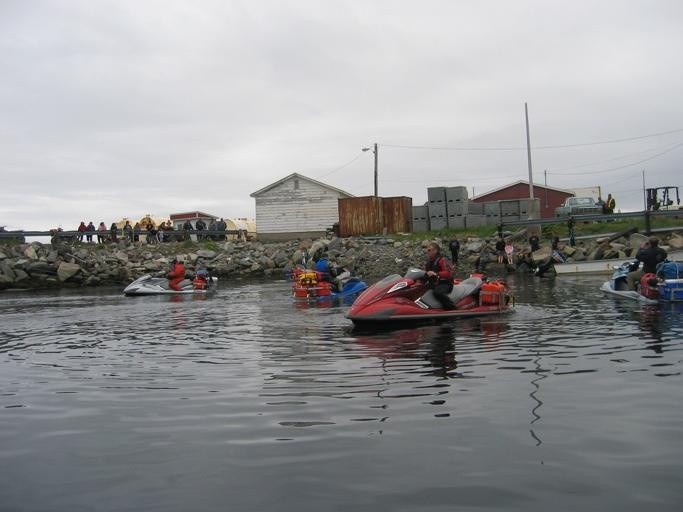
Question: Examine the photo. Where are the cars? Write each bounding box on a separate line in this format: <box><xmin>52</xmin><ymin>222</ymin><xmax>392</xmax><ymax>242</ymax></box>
<box><xmin>552</xmin><ymin>196</ymin><xmax>603</xmax><ymax>218</ymax></box>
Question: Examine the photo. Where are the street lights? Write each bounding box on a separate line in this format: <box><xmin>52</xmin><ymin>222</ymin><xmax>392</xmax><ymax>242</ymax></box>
<box><xmin>362</xmin><ymin>142</ymin><xmax>378</xmax><ymax>196</ymax></box>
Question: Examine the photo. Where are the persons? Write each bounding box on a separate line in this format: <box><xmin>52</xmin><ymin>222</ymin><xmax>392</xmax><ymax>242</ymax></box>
<box><xmin>308</xmin><ymin>255</ymin><xmax>319</xmax><ymax>269</ymax></box>
<box><xmin>425</xmin><ymin>242</ymin><xmax>457</xmax><ymax>310</ymax></box>
<box><xmin>551</xmin><ymin>232</ymin><xmax>559</xmax><ymax>249</ymax></box>
<box><xmin>165</xmin><ymin>256</ymin><xmax>186</xmax><ymax>291</ymax></box>
<box><xmin>529</xmin><ymin>232</ymin><xmax>540</xmax><ymax>251</ymax></box>
<box><xmin>504</xmin><ymin>240</ymin><xmax>514</xmax><ymax>264</ymax></box>
<box><xmin>604</xmin><ymin>193</ymin><xmax>615</xmax><ymax>213</ymax></box>
<box><xmin>496</xmin><ymin>223</ymin><xmax>504</xmax><ymax>238</ymax></box>
<box><xmin>568</xmin><ymin>217</ymin><xmax>576</xmax><ymax>246</ymax></box>
<box><xmin>595</xmin><ymin>197</ymin><xmax>607</xmax><ymax>213</ymax></box>
<box><xmin>316</xmin><ymin>252</ymin><xmax>344</xmax><ymax>293</ymax></box>
<box><xmin>495</xmin><ymin>237</ymin><xmax>506</xmax><ymax>264</ymax></box>
<box><xmin>77</xmin><ymin>218</ymin><xmax>227</xmax><ymax>245</ymax></box>
<box><xmin>449</xmin><ymin>236</ymin><xmax>460</xmax><ymax>266</ymax></box>
<box><xmin>627</xmin><ymin>238</ymin><xmax>667</xmax><ymax>290</ymax></box>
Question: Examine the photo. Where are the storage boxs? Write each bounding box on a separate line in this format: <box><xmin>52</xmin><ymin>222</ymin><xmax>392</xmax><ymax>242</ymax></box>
<box><xmin>663</xmin><ymin>277</ymin><xmax>682</xmax><ymax>301</ymax></box>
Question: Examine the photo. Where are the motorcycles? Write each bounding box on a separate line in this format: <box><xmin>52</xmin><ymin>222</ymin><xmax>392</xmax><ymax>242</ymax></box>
<box><xmin>120</xmin><ymin>268</ymin><xmax>219</xmax><ymax>296</ymax></box>
<box><xmin>283</xmin><ymin>254</ymin><xmax>369</xmax><ymax>304</ymax></box>
<box><xmin>336</xmin><ymin>265</ymin><xmax>518</xmax><ymax>328</ymax></box>
<box><xmin>595</xmin><ymin>255</ymin><xmax>682</xmax><ymax>304</ymax></box>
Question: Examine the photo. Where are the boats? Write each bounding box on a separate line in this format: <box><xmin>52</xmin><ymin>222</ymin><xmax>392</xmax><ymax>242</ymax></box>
<box><xmin>553</xmin><ymin>248</ymin><xmax>682</xmax><ymax>277</ymax></box>
